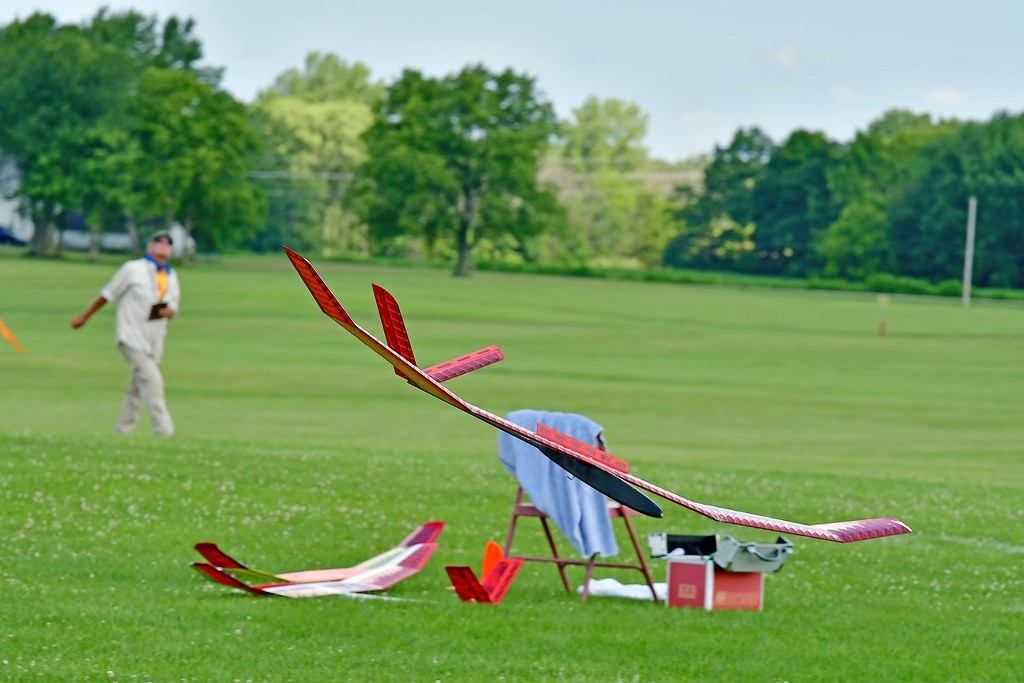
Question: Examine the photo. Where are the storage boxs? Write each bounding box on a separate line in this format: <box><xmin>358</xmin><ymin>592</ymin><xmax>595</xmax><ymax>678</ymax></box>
<box><xmin>648</xmin><ymin>531</ymin><xmax>765</xmax><ymax>612</ymax></box>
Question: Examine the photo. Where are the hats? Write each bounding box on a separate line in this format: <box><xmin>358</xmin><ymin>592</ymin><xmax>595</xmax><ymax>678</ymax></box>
<box><xmin>148</xmin><ymin>230</ymin><xmax>176</xmax><ymax>242</ymax></box>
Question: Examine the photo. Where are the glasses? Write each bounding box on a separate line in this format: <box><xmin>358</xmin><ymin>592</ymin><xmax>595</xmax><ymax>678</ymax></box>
<box><xmin>155</xmin><ymin>238</ymin><xmax>172</xmax><ymax>244</ymax></box>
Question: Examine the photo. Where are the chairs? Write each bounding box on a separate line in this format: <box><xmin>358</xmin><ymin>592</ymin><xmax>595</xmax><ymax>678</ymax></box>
<box><xmin>499</xmin><ymin>409</ymin><xmax>659</xmax><ymax>604</ymax></box>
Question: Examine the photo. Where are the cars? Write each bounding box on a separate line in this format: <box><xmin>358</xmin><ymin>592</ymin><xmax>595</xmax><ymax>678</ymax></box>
<box><xmin>169</xmin><ymin>224</ymin><xmax>200</xmax><ymax>261</ymax></box>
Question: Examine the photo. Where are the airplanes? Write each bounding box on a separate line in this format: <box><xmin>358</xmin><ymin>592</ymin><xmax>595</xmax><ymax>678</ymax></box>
<box><xmin>283</xmin><ymin>244</ymin><xmax>914</xmax><ymax>543</ymax></box>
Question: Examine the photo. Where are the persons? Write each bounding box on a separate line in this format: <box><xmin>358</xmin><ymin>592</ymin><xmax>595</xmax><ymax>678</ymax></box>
<box><xmin>72</xmin><ymin>233</ymin><xmax>180</xmax><ymax>436</ymax></box>
